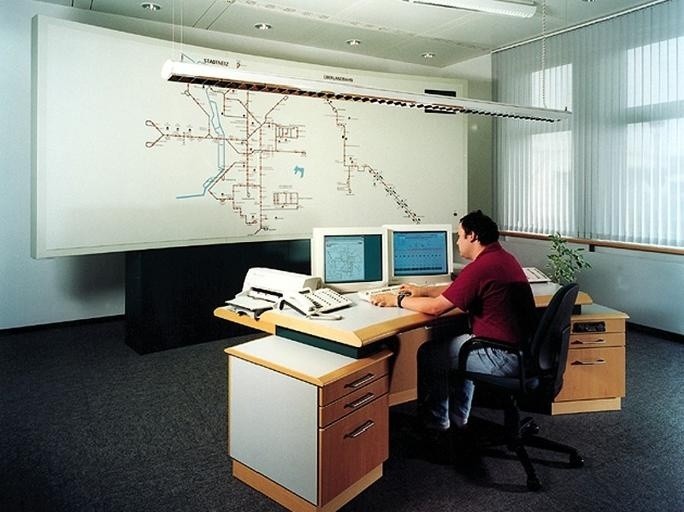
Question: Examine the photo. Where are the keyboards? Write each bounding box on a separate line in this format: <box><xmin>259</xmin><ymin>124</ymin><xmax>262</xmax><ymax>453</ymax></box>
<box><xmin>357</xmin><ymin>282</ymin><xmax>419</xmax><ymax>303</ymax></box>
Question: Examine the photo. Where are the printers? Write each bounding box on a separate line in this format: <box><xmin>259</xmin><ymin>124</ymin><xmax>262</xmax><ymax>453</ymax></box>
<box><xmin>225</xmin><ymin>266</ymin><xmax>323</xmax><ymax>321</ymax></box>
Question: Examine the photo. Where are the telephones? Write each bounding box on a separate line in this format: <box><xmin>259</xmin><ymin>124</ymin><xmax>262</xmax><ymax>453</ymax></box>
<box><xmin>283</xmin><ymin>287</ymin><xmax>353</xmax><ymax>316</ymax></box>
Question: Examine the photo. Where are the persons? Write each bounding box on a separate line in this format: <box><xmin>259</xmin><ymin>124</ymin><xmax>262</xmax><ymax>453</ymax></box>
<box><xmin>369</xmin><ymin>209</ymin><xmax>539</xmax><ymax>430</ymax></box>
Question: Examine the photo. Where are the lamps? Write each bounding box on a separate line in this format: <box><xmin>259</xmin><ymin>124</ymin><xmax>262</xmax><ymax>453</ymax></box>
<box><xmin>159</xmin><ymin>1</ymin><xmax>573</xmax><ymax>128</ymax></box>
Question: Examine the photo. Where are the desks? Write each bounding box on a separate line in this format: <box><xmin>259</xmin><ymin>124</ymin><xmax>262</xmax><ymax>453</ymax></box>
<box><xmin>212</xmin><ymin>283</ymin><xmax>631</xmax><ymax>512</ymax></box>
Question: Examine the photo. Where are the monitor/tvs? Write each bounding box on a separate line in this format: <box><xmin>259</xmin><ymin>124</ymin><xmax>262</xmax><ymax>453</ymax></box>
<box><xmin>382</xmin><ymin>223</ymin><xmax>454</xmax><ymax>285</ymax></box>
<box><xmin>310</xmin><ymin>226</ymin><xmax>389</xmax><ymax>293</ymax></box>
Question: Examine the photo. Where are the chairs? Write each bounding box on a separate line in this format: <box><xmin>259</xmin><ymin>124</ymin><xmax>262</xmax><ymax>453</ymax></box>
<box><xmin>447</xmin><ymin>283</ymin><xmax>584</xmax><ymax>492</ymax></box>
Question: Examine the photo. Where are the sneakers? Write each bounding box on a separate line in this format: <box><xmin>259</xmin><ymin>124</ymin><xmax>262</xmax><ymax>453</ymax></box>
<box><xmin>425</xmin><ymin>412</ymin><xmax>468</xmax><ymax>430</ymax></box>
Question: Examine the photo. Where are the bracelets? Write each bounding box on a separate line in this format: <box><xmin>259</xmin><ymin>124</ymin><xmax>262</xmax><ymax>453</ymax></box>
<box><xmin>397</xmin><ymin>294</ymin><xmax>406</xmax><ymax>309</ymax></box>
